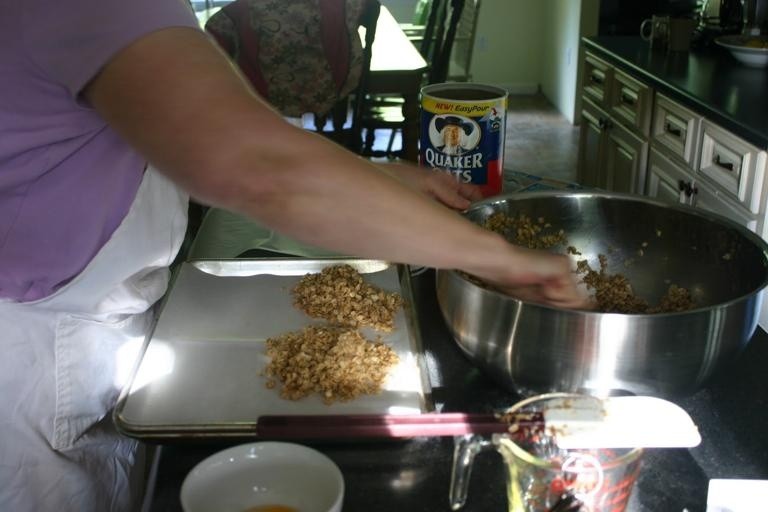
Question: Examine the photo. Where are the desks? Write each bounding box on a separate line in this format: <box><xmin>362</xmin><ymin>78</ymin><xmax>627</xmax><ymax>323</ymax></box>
<box><xmin>190</xmin><ymin>1</ymin><xmax>429</xmax><ymax>164</ymax></box>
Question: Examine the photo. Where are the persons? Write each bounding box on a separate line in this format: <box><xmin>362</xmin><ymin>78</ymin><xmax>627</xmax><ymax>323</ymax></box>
<box><xmin>0</xmin><ymin>1</ymin><xmax>599</xmax><ymax>511</ymax></box>
<box><xmin>434</xmin><ymin>116</ymin><xmax>474</xmax><ymax>155</ymax></box>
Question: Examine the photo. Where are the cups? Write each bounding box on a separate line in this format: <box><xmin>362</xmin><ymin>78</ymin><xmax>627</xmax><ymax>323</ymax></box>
<box><xmin>638</xmin><ymin>12</ymin><xmax>670</xmax><ymax>49</ymax></box>
<box><xmin>445</xmin><ymin>390</ymin><xmax>645</xmax><ymax>512</ymax></box>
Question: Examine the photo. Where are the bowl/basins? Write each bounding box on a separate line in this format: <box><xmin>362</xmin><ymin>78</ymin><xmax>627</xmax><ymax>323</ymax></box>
<box><xmin>180</xmin><ymin>440</ymin><xmax>347</xmax><ymax>512</ymax></box>
<box><xmin>433</xmin><ymin>187</ymin><xmax>762</xmax><ymax>397</ymax></box>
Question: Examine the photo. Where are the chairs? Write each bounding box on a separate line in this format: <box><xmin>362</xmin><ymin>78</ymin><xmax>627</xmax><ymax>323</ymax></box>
<box><xmin>242</xmin><ymin>1</ymin><xmax>380</xmax><ymax>152</ymax></box>
<box><xmin>356</xmin><ymin>1</ymin><xmax>465</xmax><ymax>163</ymax></box>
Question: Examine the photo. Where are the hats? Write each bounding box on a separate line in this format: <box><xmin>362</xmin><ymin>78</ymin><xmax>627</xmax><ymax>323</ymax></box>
<box><xmin>435</xmin><ymin>116</ymin><xmax>474</xmax><ymax>135</ymax></box>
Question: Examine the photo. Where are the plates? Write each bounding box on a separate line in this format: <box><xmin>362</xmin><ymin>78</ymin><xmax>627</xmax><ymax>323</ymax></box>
<box><xmin>712</xmin><ymin>34</ymin><xmax>768</xmax><ymax>67</ymax></box>
